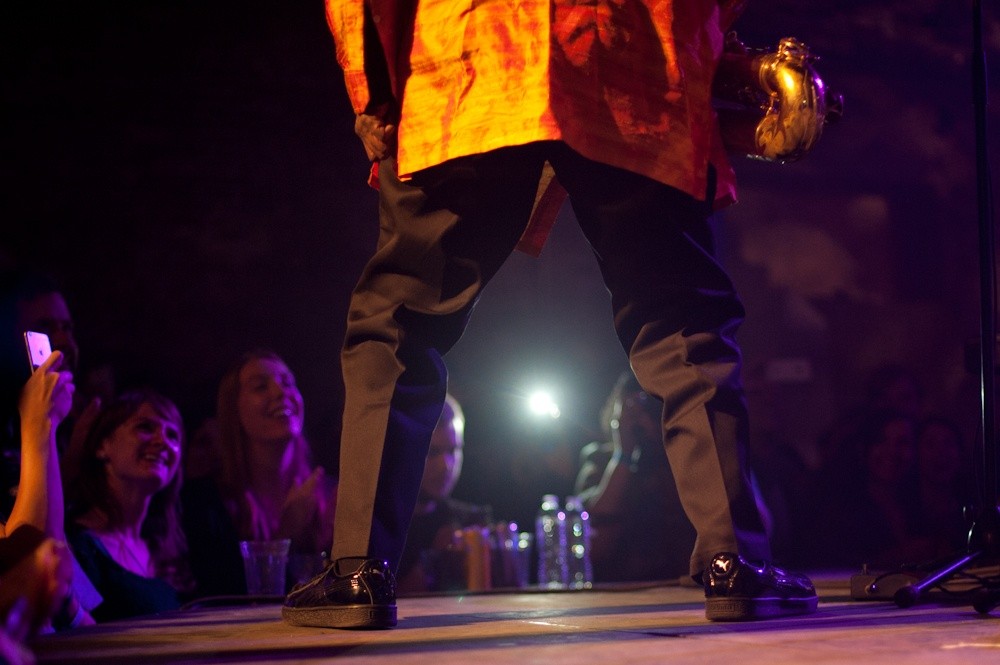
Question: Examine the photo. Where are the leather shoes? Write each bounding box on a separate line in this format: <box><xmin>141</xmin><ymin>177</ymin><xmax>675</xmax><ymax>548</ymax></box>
<box><xmin>282</xmin><ymin>561</ymin><xmax>399</xmax><ymax>628</ymax></box>
<box><xmin>704</xmin><ymin>550</ymin><xmax>819</xmax><ymax>621</ymax></box>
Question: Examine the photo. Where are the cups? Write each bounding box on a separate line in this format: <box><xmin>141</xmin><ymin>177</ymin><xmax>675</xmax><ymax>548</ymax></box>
<box><xmin>239</xmin><ymin>540</ymin><xmax>290</xmax><ymax>594</ymax></box>
<box><xmin>495</xmin><ymin>533</ymin><xmax>530</xmax><ymax>586</ymax></box>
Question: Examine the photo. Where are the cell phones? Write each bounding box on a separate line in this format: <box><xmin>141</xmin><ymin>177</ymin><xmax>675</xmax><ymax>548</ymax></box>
<box><xmin>22</xmin><ymin>329</ymin><xmax>54</xmax><ymax>376</ymax></box>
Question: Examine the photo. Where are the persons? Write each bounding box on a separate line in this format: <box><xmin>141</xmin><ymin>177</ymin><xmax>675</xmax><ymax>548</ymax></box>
<box><xmin>0</xmin><ymin>275</ymin><xmax>1000</xmax><ymax>665</ymax></box>
<box><xmin>282</xmin><ymin>0</ymin><xmax>821</xmax><ymax>628</ymax></box>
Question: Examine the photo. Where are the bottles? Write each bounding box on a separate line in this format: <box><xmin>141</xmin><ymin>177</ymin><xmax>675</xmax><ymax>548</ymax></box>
<box><xmin>536</xmin><ymin>495</ymin><xmax>568</xmax><ymax>588</ymax></box>
<box><xmin>563</xmin><ymin>496</ymin><xmax>593</xmax><ymax>589</ymax></box>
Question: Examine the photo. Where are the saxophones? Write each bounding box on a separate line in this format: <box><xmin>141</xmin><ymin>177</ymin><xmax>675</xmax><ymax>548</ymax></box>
<box><xmin>715</xmin><ymin>23</ymin><xmax>846</xmax><ymax>169</ymax></box>
